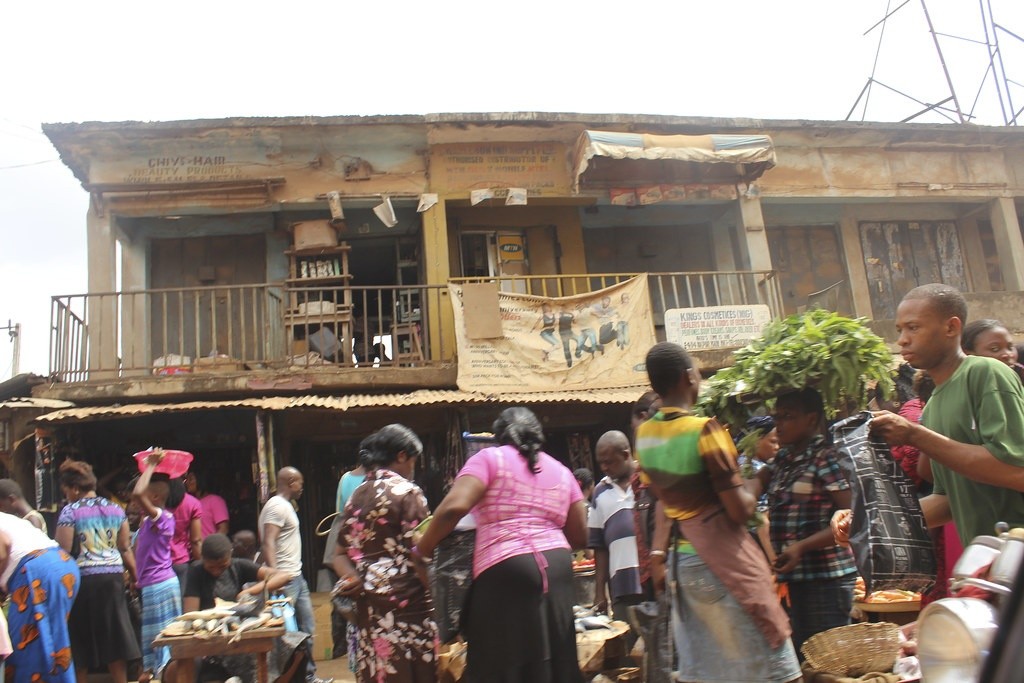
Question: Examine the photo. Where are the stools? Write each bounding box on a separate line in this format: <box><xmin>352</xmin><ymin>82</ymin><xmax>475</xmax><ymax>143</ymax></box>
<box><xmin>388</xmin><ymin>323</ymin><xmax>423</xmax><ymax>368</ymax></box>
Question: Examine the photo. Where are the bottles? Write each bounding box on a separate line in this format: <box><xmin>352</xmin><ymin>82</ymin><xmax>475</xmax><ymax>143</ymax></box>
<box><xmin>951</xmin><ymin>528</ymin><xmax>1024</xmax><ymax>586</ymax></box>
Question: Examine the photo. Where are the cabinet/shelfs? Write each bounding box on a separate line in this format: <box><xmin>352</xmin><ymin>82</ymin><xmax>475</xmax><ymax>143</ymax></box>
<box><xmin>284</xmin><ymin>239</ymin><xmax>355</xmax><ymax>370</ymax></box>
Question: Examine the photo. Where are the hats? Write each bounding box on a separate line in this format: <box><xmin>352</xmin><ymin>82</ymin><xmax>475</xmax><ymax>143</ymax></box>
<box><xmin>733</xmin><ymin>416</ymin><xmax>778</xmax><ymax>444</ymax></box>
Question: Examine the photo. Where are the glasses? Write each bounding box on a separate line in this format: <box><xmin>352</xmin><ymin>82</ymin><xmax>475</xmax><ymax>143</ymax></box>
<box><xmin>770</xmin><ymin>413</ymin><xmax>796</xmax><ymax>421</ymax></box>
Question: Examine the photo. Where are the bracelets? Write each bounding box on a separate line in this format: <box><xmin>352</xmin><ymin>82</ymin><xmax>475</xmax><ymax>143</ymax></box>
<box><xmin>412</xmin><ymin>547</ymin><xmax>423</xmax><ymax>556</ymax></box>
<box><xmin>649</xmin><ymin>549</ymin><xmax>667</xmax><ymax>558</ymax></box>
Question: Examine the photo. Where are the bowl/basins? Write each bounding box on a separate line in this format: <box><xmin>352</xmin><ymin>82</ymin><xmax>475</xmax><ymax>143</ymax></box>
<box><xmin>133</xmin><ymin>450</ymin><xmax>193</xmax><ymax>479</ymax></box>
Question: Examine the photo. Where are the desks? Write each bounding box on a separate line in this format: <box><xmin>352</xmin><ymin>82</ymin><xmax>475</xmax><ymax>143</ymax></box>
<box><xmin>152</xmin><ymin>617</ymin><xmax>286</xmax><ymax>683</ymax></box>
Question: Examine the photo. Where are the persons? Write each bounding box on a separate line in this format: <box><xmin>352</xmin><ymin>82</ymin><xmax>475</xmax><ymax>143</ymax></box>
<box><xmin>257</xmin><ymin>466</ymin><xmax>333</xmax><ymax>683</ymax></box>
<box><xmin>591</xmin><ymin>296</ymin><xmax>618</xmax><ymax>344</ymax></box>
<box><xmin>0</xmin><ymin>318</ymin><xmax>1024</xmax><ymax>683</ymax></box>
<box><xmin>530</xmin><ymin>303</ymin><xmax>562</xmax><ymax>362</ymax></box>
<box><xmin>614</xmin><ymin>293</ymin><xmax>636</xmax><ymax>350</ymax></box>
<box><xmin>555</xmin><ymin>303</ymin><xmax>593</xmax><ymax>367</ymax></box>
<box><xmin>407</xmin><ymin>408</ymin><xmax>590</xmax><ymax>683</ymax></box>
<box><xmin>333</xmin><ymin>422</ymin><xmax>439</xmax><ymax>683</ymax></box>
<box><xmin>639</xmin><ymin>343</ymin><xmax>804</xmax><ymax>683</ymax></box>
<box><xmin>371</xmin><ymin>344</ymin><xmax>392</xmax><ymax>367</ymax></box>
<box><xmin>832</xmin><ymin>284</ymin><xmax>1024</xmax><ymax>550</ymax></box>
<box><xmin>572</xmin><ymin>302</ymin><xmax>613</xmax><ymax>358</ymax></box>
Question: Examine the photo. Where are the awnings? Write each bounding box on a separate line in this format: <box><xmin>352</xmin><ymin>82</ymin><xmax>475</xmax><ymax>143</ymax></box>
<box><xmin>571</xmin><ymin>130</ymin><xmax>777</xmax><ymax>194</ymax></box>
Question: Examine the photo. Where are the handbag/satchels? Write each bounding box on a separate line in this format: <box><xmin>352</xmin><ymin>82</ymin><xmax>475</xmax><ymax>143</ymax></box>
<box><xmin>315</xmin><ymin>512</ymin><xmax>347</xmax><ymax>569</ymax></box>
<box><xmin>69</xmin><ymin>535</ymin><xmax>81</xmax><ymax>559</ymax></box>
<box><xmin>428</xmin><ymin>524</ymin><xmax>476</xmax><ymax>643</ymax></box>
<box><xmin>831</xmin><ymin>410</ymin><xmax>939</xmax><ymax>595</ymax></box>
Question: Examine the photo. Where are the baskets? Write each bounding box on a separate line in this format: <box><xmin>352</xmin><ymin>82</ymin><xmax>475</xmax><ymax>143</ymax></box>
<box><xmin>800</xmin><ymin>621</ymin><xmax>900</xmax><ymax>676</ymax></box>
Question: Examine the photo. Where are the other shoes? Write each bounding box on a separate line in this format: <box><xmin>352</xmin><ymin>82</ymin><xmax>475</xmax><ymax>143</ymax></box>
<box><xmin>86</xmin><ymin>662</ymin><xmax>108</xmax><ymax>674</ymax></box>
<box><xmin>138</xmin><ymin>672</ymin><xmax>151</xmax><ymax>683</ymax></box>
<box><xmin>304</xmin><ymin>674</ymin><xmax>334</xmax><ymax>683</ymax></box>
<box><xmin>126</xmin><ymin>660</ymin><xmax>142</xmax><ymax>683</ymax></box>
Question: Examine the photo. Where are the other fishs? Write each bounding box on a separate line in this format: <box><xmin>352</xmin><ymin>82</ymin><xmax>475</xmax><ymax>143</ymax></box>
<box><xmin>573</xmin><ymin>604</ymin><xmax>612</xmax><ymax>634</ymax></box>
<box><xmin>171</xmin><ymin>572</ymin><xmax>274</xmax><ymax>644</ymax></box>
